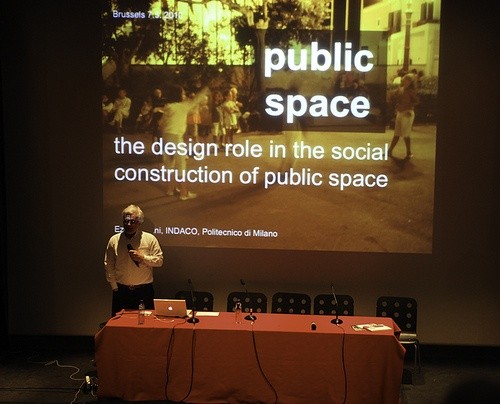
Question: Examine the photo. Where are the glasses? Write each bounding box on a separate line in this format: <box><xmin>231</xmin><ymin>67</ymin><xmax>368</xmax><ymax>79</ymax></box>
<box><xmin>124</xmin><ymin>219</ymin><xmax>137</xmax><ymax>224</ymax></box>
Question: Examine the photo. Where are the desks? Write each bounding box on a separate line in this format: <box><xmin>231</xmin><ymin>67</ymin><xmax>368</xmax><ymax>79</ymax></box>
<box><xmin>93</xmin><ymin>307</ymin><xmax>406</xmax><ymax>404</ymax></box>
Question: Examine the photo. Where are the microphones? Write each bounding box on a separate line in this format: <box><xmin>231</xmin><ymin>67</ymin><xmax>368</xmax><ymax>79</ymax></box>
<box><xmin>126</xmin><ymin>243</ymin><xmax>139</xmax><ymax>267</ymax></box>
<box><xmin>330</xmin><ymin>282</ymin><xmax>343</xmax><ymax>324</ymax></box>
<box><xmin>187</xmin><ymin>278</ymin><xmax>199</xmax><ymax>323</ymax></box>
<box><xmin>239</xmin><ymin>279</ymin><xmax>256</xmax><ymax>321</ymax></box>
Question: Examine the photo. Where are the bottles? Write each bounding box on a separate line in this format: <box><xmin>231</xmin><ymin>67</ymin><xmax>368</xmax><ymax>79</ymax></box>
<box><xmin>235</xmin><ymin>299</ymin><xmax>241</xmax><ymax>323</ymax></box>
<box><xmin>139</xmin><ymin>300</ymin><xmax>145</xmax><ymax>324</ymax></box>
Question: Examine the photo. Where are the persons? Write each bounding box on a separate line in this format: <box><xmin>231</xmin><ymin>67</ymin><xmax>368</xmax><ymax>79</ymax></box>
<box><xmin>334</xmin><ymin>70</ymin><xmax>366</xmax><ymax>102</ymax></box>
<box><xmin>378</xmin><ymin>73</ymin><xmax>416</xmax><ymax>159</ymax></box>
<box><xmin>393</xmin><ymin>69</ymin><xmax>425</xmax><ymax>88</ymax></box>
<box><xmin>158</xmin><ymin>84</ymin><xmax>208</xmax><ymax>200</ymax></box>
<box><xmin>102</xmin><ymin>88</ymin><xmax>244</xmax><ymax>152</ymax></box>
<box><xmin>104</xmin><ymin>205</ymin><xmax>164</xmax><ymax>318</ymax></box>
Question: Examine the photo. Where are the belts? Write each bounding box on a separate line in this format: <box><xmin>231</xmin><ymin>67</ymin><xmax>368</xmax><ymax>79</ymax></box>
<box><xmin>117</xmin><ymin>283</ymin><xmax>151</xmax><ymax>291</ymax></box>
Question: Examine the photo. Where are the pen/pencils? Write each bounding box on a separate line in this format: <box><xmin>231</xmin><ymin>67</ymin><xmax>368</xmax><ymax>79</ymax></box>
<box><xmin>112</xmin><ymin>315</ymin><xmax>121</xmax><ymax>320</ymax></box>
<box><xmin>374</xmin><ymin>325</ymin><xmax>384</xmax><ymax>327</ymax></box>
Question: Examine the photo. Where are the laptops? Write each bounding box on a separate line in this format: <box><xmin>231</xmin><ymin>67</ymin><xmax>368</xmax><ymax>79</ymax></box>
<box><xmin>153</xmin><ymin>298</ymin><xmax>192</xmax><ymax>318</ymax></box>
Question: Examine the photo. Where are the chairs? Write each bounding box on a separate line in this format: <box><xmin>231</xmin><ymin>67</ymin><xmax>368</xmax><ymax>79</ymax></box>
<box><xmin>174</xmin><ymin>290</ymin><xmax>214</xmax><ymax>312</ymax></box>
<box><xmin>376</xmin><ymin>296</ymin><xmax>422</xmax><ymax>387</ymax></box>
<box><xmin>313</xmin><ymin>293</ymin><xmax>355</xmax><ymax>316</ymax></box>
<box><xmin>227</xmin><ymin>292</ymin><xmax>268</xmax><ymax>313</ymax></box>
<box><xmin>271</xmin><ymin>292</ymin><xmax>312</xmax><ymax>314</ymax></box>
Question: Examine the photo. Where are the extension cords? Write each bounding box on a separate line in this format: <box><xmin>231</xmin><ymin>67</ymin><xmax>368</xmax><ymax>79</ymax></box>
<box><xmin>86</xmin><ymin>375</ymin><xmax>91</xmax><ymax>389</ymax></box>
